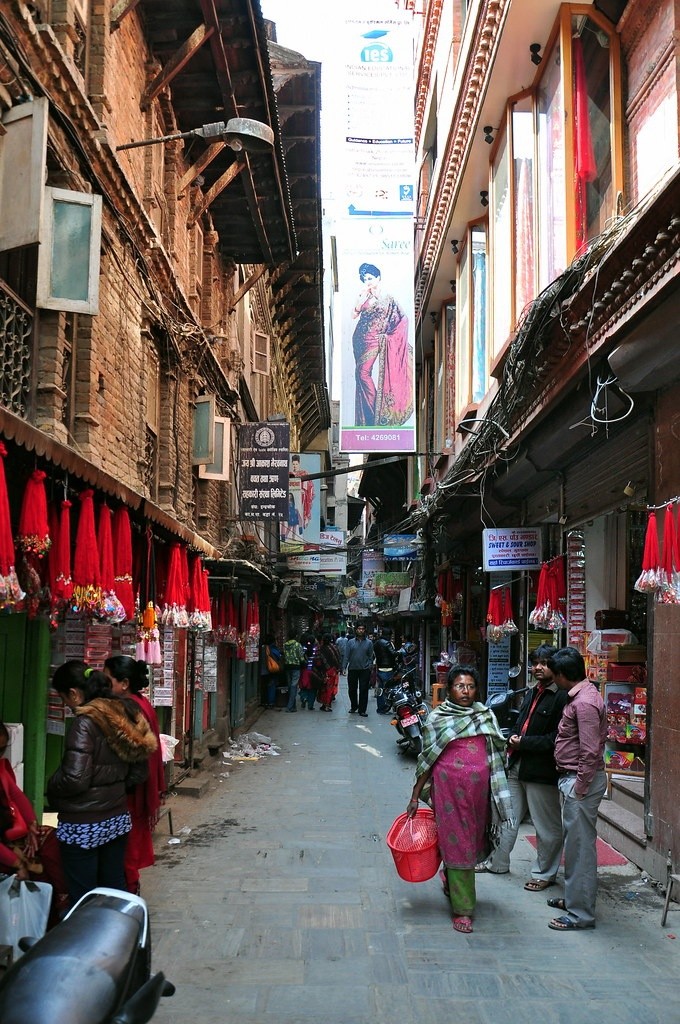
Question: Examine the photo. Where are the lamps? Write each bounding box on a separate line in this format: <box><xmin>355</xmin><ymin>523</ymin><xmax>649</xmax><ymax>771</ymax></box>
<box><xmin>450</xmin><ymin>279</ymin><xmax>457</xmax><ymax>293</ymax></box>
<box><xmin>529</xmin><ymin>43</ymin><xmax>543</xmax><ymax>66</ymax></box>
<box><xmin>480</xmin><ymin>190</ymin><xmax>488</xmax><ymax>206</ymax></box>
<box><xmin>116</xmin><ymin>117</ymin><xmax>275</xmax><ymax>155</ymax></box>
<box><xmin>484</xmin><ymin>125</ymin><xmax>494</xmax><ymax>144</ymax></box>
<box><xmin>430</xmin><ymin>312</ymin><xmax>436</xmax><ymax>323</ymax></box>
<box><xmin>450</xmin><ymin>240</ymin><xmax>459</xmax><ymax>254</ymax></box>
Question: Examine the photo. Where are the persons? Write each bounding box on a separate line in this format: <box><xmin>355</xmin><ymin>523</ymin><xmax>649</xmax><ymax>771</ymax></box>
<box><xmin>257</xmin><ymin>622</ymin><xmax>419</xmax><ymax>716</ymax></box>
<box><xmin>0</xmin><ymin>655</ymin><xmax>164</xmax><ymax>933</ymax></box>
<box><xmin>546</xmin><ymin>649</ymin><xmax>608</xmax><ymax>931</ymax></box>
<box><xmin>351</xmin><ymin>263</ymin><xmax>413</xmax><ymax>426</ymax></box>
<box><xmin>474</xmin><ymin>644</ymin><xmax>570</xmax><ymax>895</ymax></box>
<box><xmin>281</xmin><ymin>455</ymin><xmax>314</xmax><ymax>541</ymax></box>
<box><xmin>404</xmin><ymin>665</ymin><xmax>504</xmax><ymax>933</ymax></box>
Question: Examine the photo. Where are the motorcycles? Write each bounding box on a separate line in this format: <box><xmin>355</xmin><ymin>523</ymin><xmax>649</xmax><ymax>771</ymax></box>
<box><xmin>377</xmin><ymin>640</ymin><xmax>428</xmax><ymax>758</ymax></box>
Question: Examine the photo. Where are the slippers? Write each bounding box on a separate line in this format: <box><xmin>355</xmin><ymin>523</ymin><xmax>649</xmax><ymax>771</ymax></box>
<box><xmin>547</xmin><ymin>897</ymin><xmax>566</xmax><ymax>910</ymax></box>
<box><xmin>524</xmin><ymin>878</ymin><xmax>549</xmax><ymax>890</ymax></box>
<box><xmin>474</xmin><ymin>863</ymin><xmax>488</xmax><ymax>872</ymax></box>
<box><xmin>548</xmin><ymin>916</ymin><xmax>596</xmax><ymax>930</ymax></box>
<box><xmin>452</xmin><ymin>917</ymin><xmax>473</xmax><ymax>933</ymax></box>
<box><xmin>438</xmin><ymin>869</ymin><xmax>449</xmax><ymax>897</ymax></box>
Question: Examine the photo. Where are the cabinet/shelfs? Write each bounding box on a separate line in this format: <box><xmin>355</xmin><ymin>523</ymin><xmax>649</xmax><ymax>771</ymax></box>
<box><xmin>600</xmin><ymin>680</ymin><xmax>645</xmax><ymax>777</ymax></box>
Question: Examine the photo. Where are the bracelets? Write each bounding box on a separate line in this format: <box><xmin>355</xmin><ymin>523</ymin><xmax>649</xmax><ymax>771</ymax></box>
<box><xmin>354</xmin><ymin>307</ymin><xmax>360</xmax><ymax>313</ymax></box>
<box><xmin>411</xmin><ymin>799</ymin><xmax>417</xmax><ymax>802</ymax></box>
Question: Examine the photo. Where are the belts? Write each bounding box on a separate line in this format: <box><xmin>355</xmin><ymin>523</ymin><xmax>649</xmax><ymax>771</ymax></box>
<box><xmin>559</xmin><ymin>770</ymin><xmax>577</xmax><ymax>775</ymax></box>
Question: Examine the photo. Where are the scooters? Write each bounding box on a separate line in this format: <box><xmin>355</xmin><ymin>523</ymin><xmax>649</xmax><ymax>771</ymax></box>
<box><xmin>484</xmin><ymin>664</ymin><xmax>529</xmax><ymax>778</ymax></box>
<box><xmin>0</xmin><ymin>886</ymin><xmax>177</xmax><ymax>1024</ymax></box>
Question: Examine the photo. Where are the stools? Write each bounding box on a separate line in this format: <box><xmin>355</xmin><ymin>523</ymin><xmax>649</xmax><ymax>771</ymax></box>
<box><xmin>431</xmin><ymin>683</ymin><xmax>447</xmax><ymax>709</ymax></box>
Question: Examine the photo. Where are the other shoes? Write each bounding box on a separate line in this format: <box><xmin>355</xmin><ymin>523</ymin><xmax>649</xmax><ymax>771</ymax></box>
<box><xmin>359</xmin><ymin>712</ymin><xmax>369</xmax><ymax>717</ymax></box>
<box><xmin>308</xmin><ymin>706</ymin><xmax>315</xmax><ymax>710</ymax></box>
<box><xmin>319</xmin><ymin>705</ymin><xmax>332</xmax><ymax>712</ymax></box>
<box><xmin>349</xmin><ymin>705</ymin><xmax>358</xmax><ymax>713</ymax></box>
<box><xmin>286</xmin><ymin>707</ymin><xmax>296</xmax><ymax>712</ymax></box>
<box><xmin>301</xmin><ymin>700</ymin><xmax>306</xmax><ymax>708</ymax></box>
<box><xmin>380</xmin><ymin>711</ymin><xmax>392</xmax><ymax>715</ymax></box>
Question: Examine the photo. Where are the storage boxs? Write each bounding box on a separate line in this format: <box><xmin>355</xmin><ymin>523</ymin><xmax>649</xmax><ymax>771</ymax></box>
<box><xmin>605</xmin><ymin>645</ymin><xmax>649</xmax><ymax>768</ymax></box>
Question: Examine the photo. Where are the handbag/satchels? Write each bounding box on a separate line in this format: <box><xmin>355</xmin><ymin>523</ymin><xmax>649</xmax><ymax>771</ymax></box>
<box><xmin>0</xmin><ymin>873</ymin><xmax>53</xmax><ymax>965</ymax></box>
<box><xmin>266</xmin><ymin>645</ymin><xmax>280</xmax><ymax>673</ymax></box>
